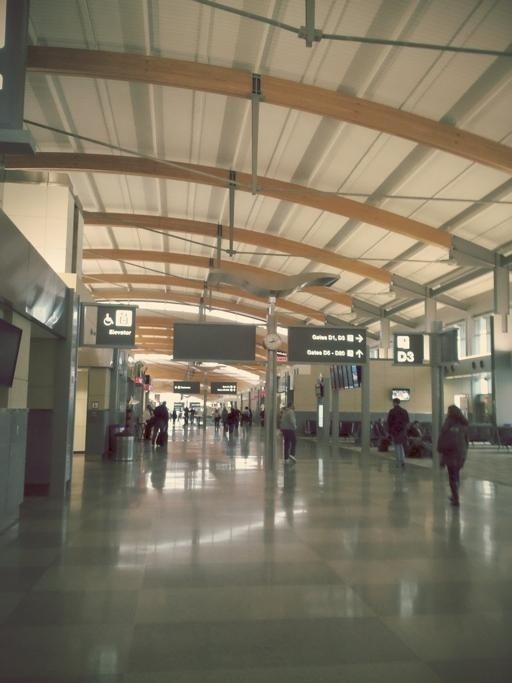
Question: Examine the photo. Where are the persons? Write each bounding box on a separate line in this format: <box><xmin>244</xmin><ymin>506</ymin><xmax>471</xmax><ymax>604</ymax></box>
<box><xmin>171</xmin><ymin>424</ymin><xmax>251</xmax><ymax>459</ymax></box>
<box><xmin>144</xmin><ymin>404</ymin><xmax>155</xmax><ymax>439</ymax></box>
<box><xmin>388</xmin><ymin>468</ymin><xmax>411</xmax><ymax>533</ymax></box>
<box><xmin>280</xmin><ymin>401</ymin><xmax>298</xmax><ymax>465</ymax></box>
<box><xmin>142</xmin><ymin>440</ymin><xmax>168</xmax><ymax>494</ymax></box>
<box><xmin>473</xmin><ymin>392</ymin><xmax>488</xmax><ymax>424</ymax></box>
<box><xmin>280</xmin><ymin>461</ymin><xmax>295</xmax><ymax>527</ymax></box>
<box><xmin>436</xmin><ymin>404</ymin><xmax>469</xmax><ymax>508</ymax></box>
<box><xmin>213</xmin><ymin>404</ymin><xmax>251</xmax><ymax>435</ymax></box>
<box><xmin>373</xmin><ymin>417</ymin><xmax>388</xmax><ymax>435</ymax></box>
<box><xmin>389</xmin><ymin>398</ymin><xmax>409</xmax><ymax>468</ymax></box>
<box><xmin>172</xmin><ymin>406</ymin><xmax>203</xmax><ymax>425</ymax></box>
<box><xmin>407</xmin><ymin>420</ymin><xmax>427</xmax><ymax>440</ymax></box>
<box><xmin>152</xmin><ymin>399</ymin><xmax>170</xmax><ymax>443</ymax></box>
<box><xmin>435</xmin><ymin>502</ymin><xmax>474</xmax><ymax>612</ymax></box>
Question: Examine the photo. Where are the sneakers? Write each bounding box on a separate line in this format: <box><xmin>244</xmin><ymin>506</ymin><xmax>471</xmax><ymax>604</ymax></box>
<box><xmin>288</xmin><ymin>454</ymin><xmax>296</xmax><ymax>461</ymax></box>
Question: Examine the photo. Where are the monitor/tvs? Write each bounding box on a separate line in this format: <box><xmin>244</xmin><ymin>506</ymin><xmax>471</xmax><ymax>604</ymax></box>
<box><xmin>391</xmin><ymin>388</ymin><xmax>410</xmax><ymax>400</ymax></box>
<box><xmin>0</xmin><ymin>319</ymin><xmax>23</xmax><ymax>388</ymax></box>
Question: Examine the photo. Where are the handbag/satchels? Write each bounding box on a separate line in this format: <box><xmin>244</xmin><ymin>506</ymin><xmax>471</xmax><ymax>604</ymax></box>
<box><xmin>437</xmin><ymin>430</ymin><xmax>458</xmax><ymax>453</ymax></box>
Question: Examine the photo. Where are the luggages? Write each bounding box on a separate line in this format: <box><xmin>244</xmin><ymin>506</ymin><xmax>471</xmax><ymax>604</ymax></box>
<box><xmin>156</xmin><ymin>424</ymin><xmax>168</xmax><ymax>445</ymax></box>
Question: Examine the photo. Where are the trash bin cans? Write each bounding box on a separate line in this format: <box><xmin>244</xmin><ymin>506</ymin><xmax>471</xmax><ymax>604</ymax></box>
<box><xmin>108</xmin><ymin>424</ymin><xmax>127</xmax><ymax>452</ymax></box>
<box><xmin>114</xmin><ymin>432</ymin><xmax>133</xmax><ymax>461</ymax></box>
